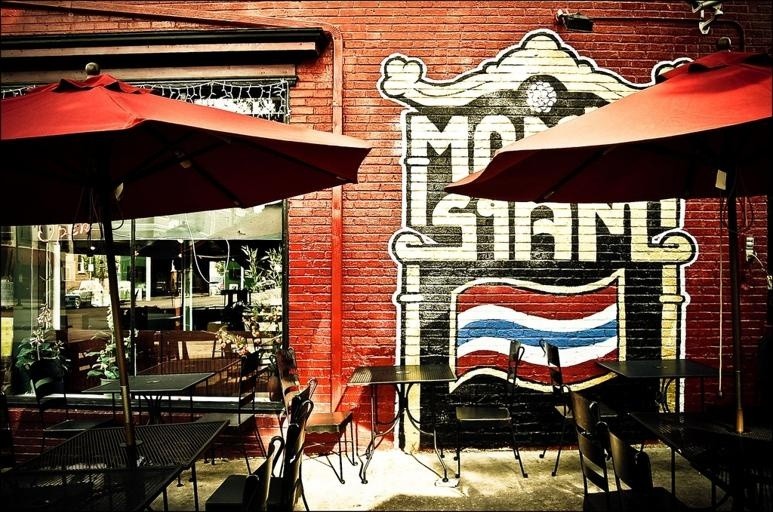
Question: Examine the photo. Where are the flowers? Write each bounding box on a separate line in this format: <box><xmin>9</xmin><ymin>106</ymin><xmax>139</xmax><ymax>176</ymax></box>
<box><xmin>15</xmin><ymin>302</ymin><xmax>67</xmax><ymax>393</ymax></box>
<box><xmin>214</xmin><ymin>325</ymin><xmax>236</xmax><ymax>349</ymax></box>
<box><xmin>85</xmin><ymin>303</ymin><xmax>140</xmax><ymax>381</ymax></box>
<box><xmin>268</xmin><ymin>309</ymin><xmax>284</xmax><ymax>376</ymax></box>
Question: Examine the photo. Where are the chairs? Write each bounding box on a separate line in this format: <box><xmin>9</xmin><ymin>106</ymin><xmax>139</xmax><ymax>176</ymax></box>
<box><xmin>543</xmin><ymin>339</ymin><xmax>618</xmax><ymax>476</ymax></box>
<box><xmin>574</xmin><ymin>420</ymin><xmax>691</xmax><ymax>512</ymax></box>
<box><xmin>1</xmin><ymin>346</ymin><xmax>358</xmax><ymax>512</ymax></box>
<box><xmin>454</xmin><ymin>340</ymin><xmax>532</xmax><ymax>480</ymax></box>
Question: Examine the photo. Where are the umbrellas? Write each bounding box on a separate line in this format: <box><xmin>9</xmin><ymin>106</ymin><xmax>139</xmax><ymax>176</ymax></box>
<box><xmin>0</xmin><ymin>61</ymin><xmax>374</xmax><ymax>447</ymax></box>
<box><xmin>440</xmin><ymin>34</ymin><xmax>773</xmax><ymax>436</ymax></box>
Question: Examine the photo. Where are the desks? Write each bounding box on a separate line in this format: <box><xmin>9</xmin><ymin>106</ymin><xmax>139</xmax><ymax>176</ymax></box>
<box><xmin>346</xmin><ymin>364</ymin><xmax>458</xmax><ymax>484</ymax></box>
<box><xmin>624</xmin><ymin>404</ymin><xmax>772</xmax><ymax>511</ymax></box>
<box><xmin>593</xmin><ymin>356</ymin><xmax>728</xmax><ymax>458</ymax></box>
